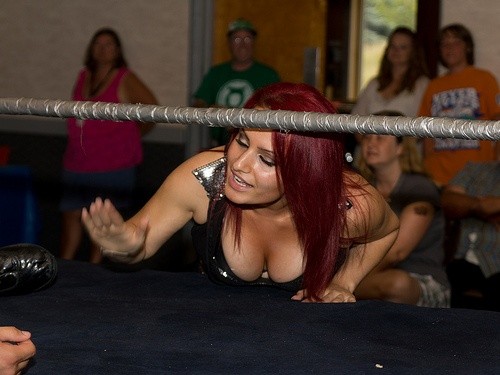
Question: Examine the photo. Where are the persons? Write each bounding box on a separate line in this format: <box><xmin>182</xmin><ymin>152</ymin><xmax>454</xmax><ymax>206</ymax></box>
<box><xmin>356</xmin><ymin>110</ymin><xmax>451</xmax><ymax>308</ymax></box>
<box><xmin>441</xmin><ymin>161</ymin><xmax>500</xmax><ymax>312</ymax></box>
<box><xmin>61</xmin><ymin>28</ymin><xmax>159</xmax><ymax>264</ymax></box>
<box><xmin>0</xmin><ymin>243</ymin><xmax>58</xmax><ymax>375</ymax></box>
<box><xmin>190</xmin><ymin>17</ymin><xmax>282</xmax><ymax>149</ymax></box>
<box><xmin>350</xmin><ymin>27</ymin><xmax>432</xmax><ymax>168</ymax></box>
<box><xmin>416</xmin><ymin>25</ymin><xmax>500</xmax><ymax>194</ymax></box>
<box><xmin>81</xmin><ymin>82</ymin><xmax>400</xmax><ymax>303</ymax></box>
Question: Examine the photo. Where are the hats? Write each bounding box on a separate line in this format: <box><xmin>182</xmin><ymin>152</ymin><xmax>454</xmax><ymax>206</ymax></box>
<box><xmin>226</xmin><ymin>18</ymin><xmax>258</xmax><ymax>36</ymax></box>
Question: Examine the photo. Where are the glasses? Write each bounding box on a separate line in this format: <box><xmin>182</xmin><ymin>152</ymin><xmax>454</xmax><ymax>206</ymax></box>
<box><xmin>231</xmin><ymin>36</ymin><xmax>252</xmax><ymax>44</ymax></box>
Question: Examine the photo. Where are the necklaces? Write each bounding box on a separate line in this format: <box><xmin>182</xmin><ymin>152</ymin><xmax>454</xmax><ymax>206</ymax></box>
<box><xmin>88</xmin><ymin>66</ymin><xmax>114</xmax><ymax>97</ymax></box>
